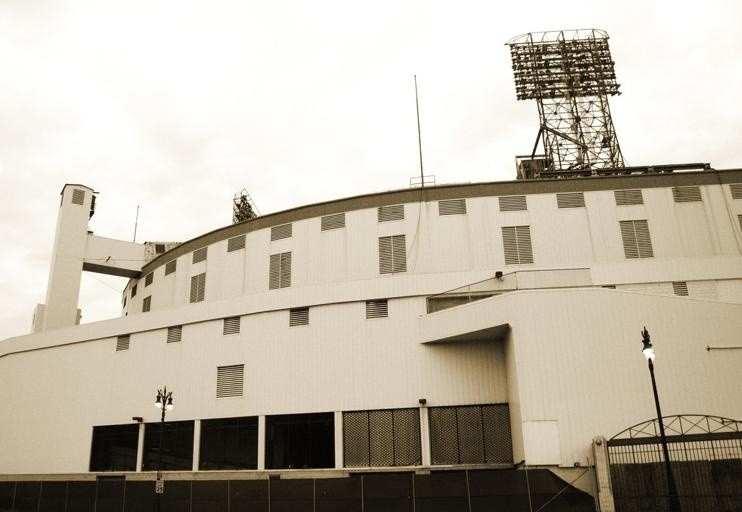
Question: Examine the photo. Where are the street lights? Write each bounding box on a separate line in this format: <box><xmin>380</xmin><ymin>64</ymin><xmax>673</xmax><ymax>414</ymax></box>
<box><xmin>233</xmin><ymin>189</ymin><xmax>262</xmax><ymax>225</ymax></box>
<box><xmin>154</xmin><ymin>386</ymin><xmax>173</xmax><ymax>511</ymax></box>
<box><xmin>639</xmin><ymin>326</ymin><xmax>681</xmax><ymax>511</ymax></box>
<box><xmin>503</xmin><ymin>27</ymin><xmax>626</xmax><ymax>179</ymax></box>
